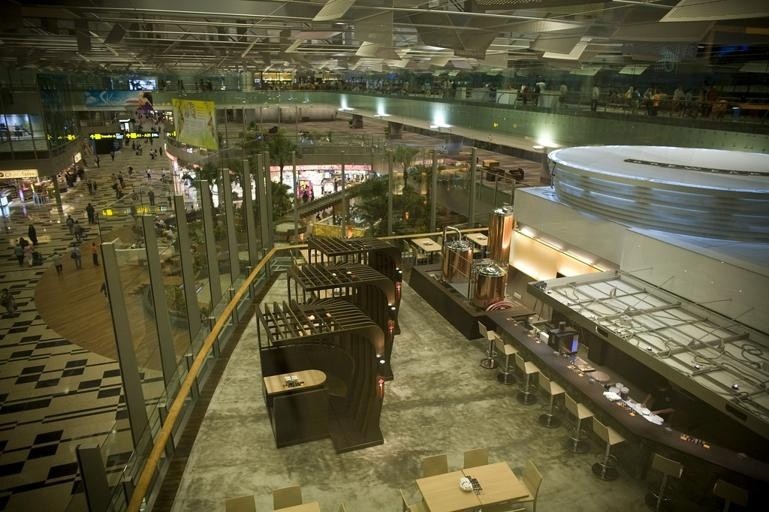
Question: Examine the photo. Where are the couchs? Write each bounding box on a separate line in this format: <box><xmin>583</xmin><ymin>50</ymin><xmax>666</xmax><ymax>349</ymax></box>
<box><xmin>259</xmin><ymin>342</ymin><xmax>354</xmax><ymax>446</ymax></box>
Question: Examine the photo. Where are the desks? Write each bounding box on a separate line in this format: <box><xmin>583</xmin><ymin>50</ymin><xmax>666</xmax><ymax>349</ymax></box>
<box><xmin>261</xmin><ymin>369</ymin><xmax>326</xmax><ymax>399</ymax></box>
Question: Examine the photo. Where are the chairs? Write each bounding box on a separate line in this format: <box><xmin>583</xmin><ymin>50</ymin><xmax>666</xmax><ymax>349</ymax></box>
<box><xmin>403</xmin><ymin>233</ymin><xmax>490</xmax><ymax>269</ymax></box>
<box><xmin>226</xmin><ymin>485</ymin><xmax>346</xmax><ymax>511</ymax></box>
<box><xmin>400</xmin><ymin>447</ymin><xmax>543</xmax><ymax>512</ymax></box>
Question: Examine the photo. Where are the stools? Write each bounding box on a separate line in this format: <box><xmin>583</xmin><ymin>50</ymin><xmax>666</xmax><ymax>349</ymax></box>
<box><xmin>494</xmin><ymin>334</ymin><xmax>519</xmax><ymax>385</ymax></box>
<box><xmin>477</xmin><ymin>320</ymin><xmax>500</xmax><ymax>370</ymax></box>
<box><xmin>590</xmin><ymin>413</ymin><xmax>626</xmax><ymax>481</ymax></box>
<box><xmin>538</xmin><ymin>369</ymin><xmax>564</xmax><ymax>429</ymax></box>
<box><xmin>565</xmin><ymin>391</ymin><xmax>594</xmax><ymax>452</ymax></box>
<box><xmin>515</xmin><ymin>351</ymin><xmax>539</xmax><ymax>405</ymax></box>
<box><xmin>645</xmin><ymin>449</ymin><xmax>683</xmax><ymax>508</ymax></box>
<box><xmin>710</xmin><ymin>473</ymin><xmax>748</xmax><ymax>508</ymax></box>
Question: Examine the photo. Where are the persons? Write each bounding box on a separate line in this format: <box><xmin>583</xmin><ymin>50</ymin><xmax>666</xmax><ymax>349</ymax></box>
<box><xmin>257</xmin><ymin>78</ymin><xmax>348</xmax><ymax>91</ymax></box>
<box><xmin>1</xmin><ymin>113</ymin><xmax>175</xmax><ymax>317</ymax></box>
<box><xmin>641</xmin><ymin>379</ymin><xmax>676</xmax><ymax>421</ymax></box>
<box><xmin>160</xmin><ymin>78</ymin><xmax>213</xmax><ymax>93</ymax></box>
<box><xmin>591</xmin><ymin>83</ymin><xmax>727</xmax><ymax>118</ymax></box>
<box><xmin>517</xmin><ymin>79</ymin><xmax>568</xmax><ymax>106</ymax></box>
<box><xmin>352</xmin><ymin>79</ymin><xmax>409</xmax><ymax>96</ymax></box>
<box><xmin>423</xmin><ymin>78</ymin><xmax>496</xmax><ymax>101</ymax></box>
<box><xmin>301</xmin><ymin>174</ymin><xmax>364</xmax><ymax>222</ymax></box>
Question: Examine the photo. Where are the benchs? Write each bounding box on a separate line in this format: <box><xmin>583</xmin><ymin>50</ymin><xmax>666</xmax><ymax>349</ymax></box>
<box><xmin>738</xmin><ymin>101</ymin><xmax>769</xmax><ymax>117</ymax></box>
<box><xmin>608</xmin><ymin>97</ymin><xmax>632</xmax><ymax>110</ymax></box>
